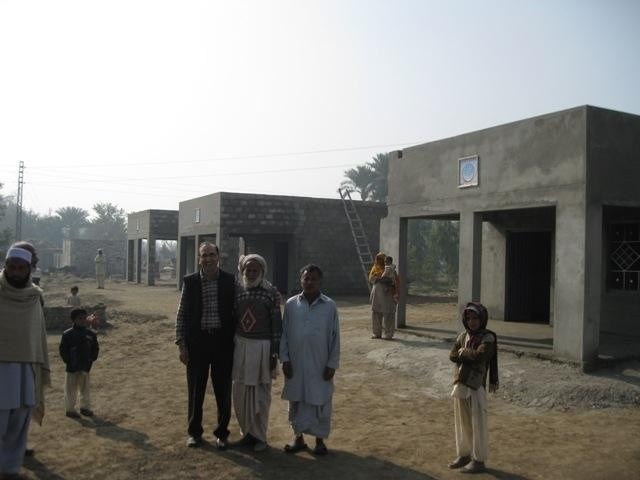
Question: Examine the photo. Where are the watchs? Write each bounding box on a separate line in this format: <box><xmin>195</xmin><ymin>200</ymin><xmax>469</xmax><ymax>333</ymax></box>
<box><xmin>271</xmin><ymin>353</ymin><xmax>279</xmax><ymax>359</ymax></box>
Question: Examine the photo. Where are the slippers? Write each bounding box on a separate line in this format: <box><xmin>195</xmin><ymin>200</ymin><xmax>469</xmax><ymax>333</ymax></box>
<box><xmin>284</xmin><ymin>441</ymin><xmax>307</xmax><ymax>454</ymax></box>
<box><xmin>314</xmin><ymin>443</ymin><xmax>328</xmax><ymax>455</ymax></box>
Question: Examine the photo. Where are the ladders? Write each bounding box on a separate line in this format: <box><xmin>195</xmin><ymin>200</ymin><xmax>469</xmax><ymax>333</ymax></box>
<box><xmin>339</xmin><ymin>188</ymin><xmax>375</xmax><ymax>295</ymax></box>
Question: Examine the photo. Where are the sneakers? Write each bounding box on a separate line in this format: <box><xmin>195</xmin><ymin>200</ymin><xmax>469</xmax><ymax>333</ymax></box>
<box><xmin>216</xmin><ymin>438</ymin><xmax>228</xmax><ymax>449</ymax></box>
<box><xmin>448</xmin><ymin>455</ymin><xmax>487</xmax><ymax>475</ymax></box>
<box><xmin>187</xmin><ymin>436</ymin><xmax>201</xmax><ymax>447</ymax></box>
<box><xmin>80</xmin><ymin>408</ymin><xmax>94</xmax><ymax>418</ymax></box>
<box><xmin>66</xmin><ymin>410</ymin><xmax>81</xmax><ymax>420</ymax></box>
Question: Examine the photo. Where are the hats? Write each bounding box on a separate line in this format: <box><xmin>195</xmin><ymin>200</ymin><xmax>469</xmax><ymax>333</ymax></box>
<box><xmin>465</xmin><ymin>305</ymin><xmax>480</xmax><ymax>316</ymax></box>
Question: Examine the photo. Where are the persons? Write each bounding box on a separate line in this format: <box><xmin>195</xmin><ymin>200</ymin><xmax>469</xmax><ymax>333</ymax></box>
<box><xmin>60</xmin><ymin>309</ymin><xmax>99</xmax><ymax>419</ymax></box>
<box><xmin>0</xmin><ymin>248</ymin><xmax>51</xmax><ymax>479</ymax></box>
<box><xmin>95</xmin><ymin>249</ymin><xmax>106</xmax><ymax>289</ymax></box>
<box><xmin>380</xmin><ymin>257</ymin><xmax>397</xmax><ymax>293</ymax></box>
<box><xmin>369</xmin><ymin>253</ymin><xmax>399</xmax><ymax>340</ymax></box>
<box><xmin>279</xmin><ymin>264</ymin><xmax>340</xmax><ymax>455</ymax></box>
<box><xmin>447</xmin><ymin>302</ymin><xmax>499</xmax><ymax>473</ymax></box>
<box><xmin>68</xmin><ymin>286</ymin><xmax>80</xmax><ymax>305</ymax></box>
<box><xmin>10</xmin><ymin>242</ymin><xmax>40</xmax><ymax>272</ymax></box>
<box><xmin>176</xmin><ymin>240</ymin><xmax>235</xmax><ymax>450</ymax></box>
<box><xmin>230</xmin><ymin>254</ymin><xmax>283</xmax><ymax>451</ymax></box>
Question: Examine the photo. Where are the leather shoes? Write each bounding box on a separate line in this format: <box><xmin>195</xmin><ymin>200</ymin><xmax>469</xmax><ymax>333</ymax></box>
<box><xmin>254</xmin><ymin>441</ymin><xmax>269</xmax><ymax>451</ymax></box>
<box><xmin>239</xmin><ymin>434</ymin><xmax>257</xmax><ymax>446</ymax></box>
